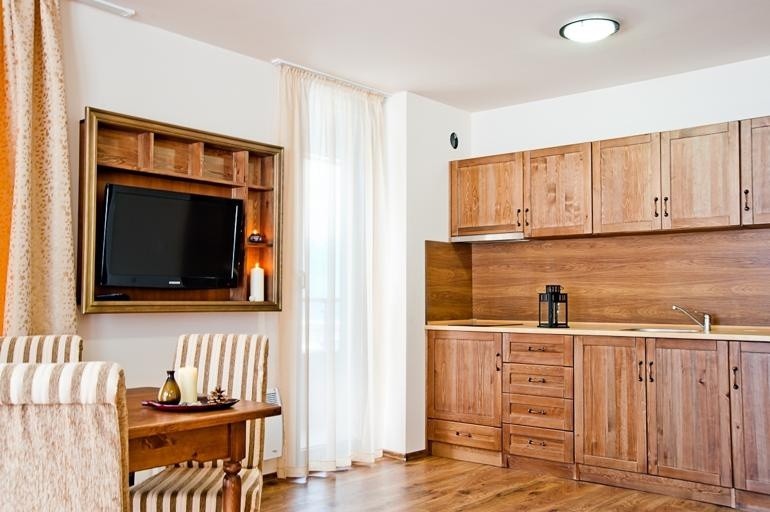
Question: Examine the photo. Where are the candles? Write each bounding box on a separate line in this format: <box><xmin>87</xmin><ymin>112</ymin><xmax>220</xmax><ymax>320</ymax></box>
<box><xmin>249</xmin><ymin>267</ymin><xmax>265</xmax><ymax>301</ymax></box>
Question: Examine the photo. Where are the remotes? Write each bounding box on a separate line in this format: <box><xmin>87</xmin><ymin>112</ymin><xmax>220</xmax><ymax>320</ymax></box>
<box><xmin>95</xmin><ymin>292</ymin><xmax>130</xmax><ymax>301</ymax></box>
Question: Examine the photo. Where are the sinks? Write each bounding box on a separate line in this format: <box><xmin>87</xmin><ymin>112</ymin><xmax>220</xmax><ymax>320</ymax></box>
<box><xmin>620</xmin><ymin>326</ymin><xmax>705</xmax><ymax>334</ymax></box>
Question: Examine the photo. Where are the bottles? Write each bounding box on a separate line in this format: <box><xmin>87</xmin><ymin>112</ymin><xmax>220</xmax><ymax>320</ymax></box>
<box><xmin>157</xmin><ymin>371</ymin><xmax>181</xmax><ymax>405</ymax></box>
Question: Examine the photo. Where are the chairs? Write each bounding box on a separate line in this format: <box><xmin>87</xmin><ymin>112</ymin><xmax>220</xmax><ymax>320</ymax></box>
<box><xmin>0</xmin><ymin>361</ymin><xmax>132</xmax><ymax>511</ymax></box>
<box><xmin>127</xmin><ymin>331</ymin><xmax>270</xmax><ymax>512</ymax></box>
<box><xmin>0</xmin><ymin>335</ymin><xmax>84</xmax><ymax>362</ymax></box>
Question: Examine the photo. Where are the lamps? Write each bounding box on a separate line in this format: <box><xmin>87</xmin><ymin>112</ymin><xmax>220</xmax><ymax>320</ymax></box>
<box><xmin>557</xmin><ymin>12</ymin><xmax>619</xmax><ymax>45</ymax></box>
<box><xmin>536</xmin><ymin>282</ymin><xmax>573</xmax><ymax>330</ymax></box>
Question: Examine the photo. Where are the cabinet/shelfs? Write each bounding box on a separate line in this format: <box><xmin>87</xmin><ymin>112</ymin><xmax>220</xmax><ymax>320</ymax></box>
<box><xmin>423</xmin><ymin>331</ymin><xmax>504</xmax><ymax>466</ymax></box>
<box><xmin>497</xmin><ymin>333</ymin><xmax>578</xmax><ymax>482</ymax></box>
<box><xmin>75</xmin><ymin>104</ymin><xmax>288</xmax><ymax>319</ymax></box>
<box><xmin>724</xmin><ymin>340</ymin><xmax>770</xmax><ymax>512</ymax></box>
<box><xmin>450</xmin><ymin>142</ymin><xmax>594</xmax><ymax>239</ymax></box>
<box><xmin>740</xmin><ymin>116</ymin><xmax>770</xmax><ymax>231</ymax></box>
<box><xmin>592</xmin><ymin>119</ymin><xmax>740</xmax><ymax>235</ymax></box>
<box><xmin>571</xmin><ymin>336</ymin><xmax>733</xmax><ymax>511</ymax></box>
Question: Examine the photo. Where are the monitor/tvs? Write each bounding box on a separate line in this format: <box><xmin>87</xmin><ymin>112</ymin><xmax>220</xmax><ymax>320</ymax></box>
<box><xmin>98</xmin><ymin>183</ymin><xmax>245</xmax><ymax>290</ymax></box>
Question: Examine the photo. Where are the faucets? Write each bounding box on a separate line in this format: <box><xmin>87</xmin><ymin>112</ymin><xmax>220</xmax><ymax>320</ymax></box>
<box><xmin>671</xmin><ymin>303</ymin><xmax>704</xmax><ymax>330</ymax></box>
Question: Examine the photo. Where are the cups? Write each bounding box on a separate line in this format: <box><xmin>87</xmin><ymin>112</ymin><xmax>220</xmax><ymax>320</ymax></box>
<box><xmin>181</xmin><ymin>367</ymin><xmax>198</xmax><ymax>402</ymax></box>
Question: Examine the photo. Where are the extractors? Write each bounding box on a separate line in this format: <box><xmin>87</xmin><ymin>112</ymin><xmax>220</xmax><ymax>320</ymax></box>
<box><xmin>449</xmin><ymin>232</ymin><xmax>529</xmax><ymax>245</ymax></box>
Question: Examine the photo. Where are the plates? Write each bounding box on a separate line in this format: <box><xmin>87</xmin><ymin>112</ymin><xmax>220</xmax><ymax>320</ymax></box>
<box><xmin>140</xmin><ymin>397</ymin><xmax>239</xmax><ymax>411</ymax></box>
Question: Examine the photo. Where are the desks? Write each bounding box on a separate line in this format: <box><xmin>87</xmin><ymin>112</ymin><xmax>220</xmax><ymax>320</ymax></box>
<box><xmin>120</xmin><ymin>385</ymin><xmax>283</xmax><ymax>512</ymax></box>
